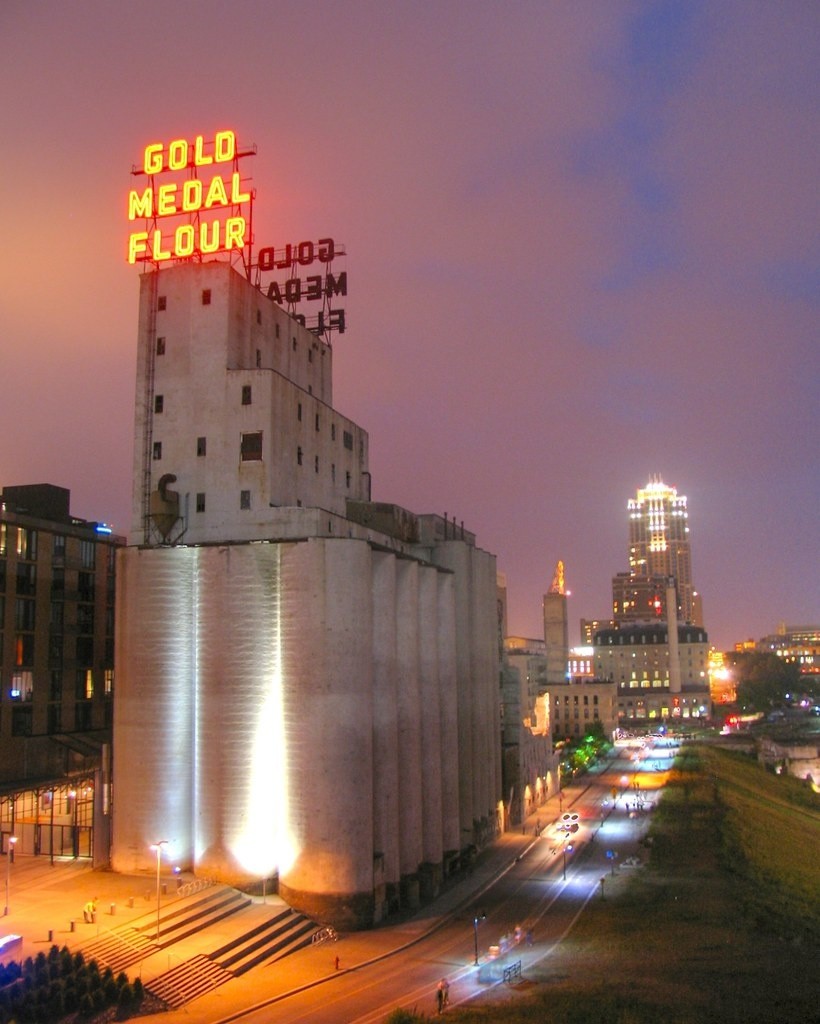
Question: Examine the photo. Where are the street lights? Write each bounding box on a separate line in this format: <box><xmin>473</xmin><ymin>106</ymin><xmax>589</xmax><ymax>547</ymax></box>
<box><xmin>4</xmin><ymin>836</ymin><xmax>20</xmax><ymax>915</ymax></box>
<box><xmin>151</xmin><ymin>840</ymin><xmax>170</xmax><ymax>937</ymax></box>
<box><xmin>563</xmin><ymin>845</ymin><xmax>572</xmax><ymax>881</ymax></box>
<box><xmin>620</xmin><ymin>776</ymin><xmax>626</xmax><ymax>799</ymax></box>
<box><xmin>473</xmin><ymin>915</ymin><xmax>486</xmax><ymax>966</ymax></box>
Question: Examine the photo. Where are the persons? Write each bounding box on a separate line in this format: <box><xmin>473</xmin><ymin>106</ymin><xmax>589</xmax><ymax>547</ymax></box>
<box><xmin>499</xmin><ymin>923</ymin><xmax>534</xmax><ymax>958</ymax></box>
<box><xmin>435</xmin><ymin>978</ymin><xmax>450</xmax><ymax>1015</ymax></box>
<box><xmin>83</xmin><ymin>896</ymin><xmax>98</xmax><ymax>923</ymax></box>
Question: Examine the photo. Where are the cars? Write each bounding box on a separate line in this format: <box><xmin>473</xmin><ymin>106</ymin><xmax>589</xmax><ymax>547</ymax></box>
<box><xmin>809</xmin><ymin>705</ymin><xmax>820</xmax><ymax>716</ymax></box>
